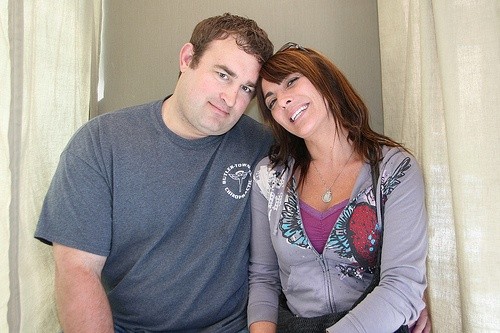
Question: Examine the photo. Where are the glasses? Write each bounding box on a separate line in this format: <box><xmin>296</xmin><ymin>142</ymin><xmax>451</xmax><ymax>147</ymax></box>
<box><xmin>274</xmin><ymin>42</ymin><xmax>310</xmax><ymax>55</ymax></box>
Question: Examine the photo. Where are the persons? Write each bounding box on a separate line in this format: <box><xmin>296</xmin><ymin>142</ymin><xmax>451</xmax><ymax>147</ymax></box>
<box><xmin>32</xmin><ymin>10</ymin><xmax>433</xmax><ymax>333</ymax></box>
<box><xmin>247</xmin><ymin>40</ymin><xmax>430</xmax><ymax>332</ymax></box>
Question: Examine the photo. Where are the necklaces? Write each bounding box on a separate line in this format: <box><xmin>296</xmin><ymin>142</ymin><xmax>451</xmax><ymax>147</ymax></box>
<box><xmin>309</xmin><ymin>143</ymin><xmax>358</xmax><ymax>203</ymax></box>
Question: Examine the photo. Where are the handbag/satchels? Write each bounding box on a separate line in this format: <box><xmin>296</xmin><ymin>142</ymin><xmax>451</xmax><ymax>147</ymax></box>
<box><xmin>278</xmin><ymin>240</ymin><xmax>410</xmax><ymax>333</ymax></box>
